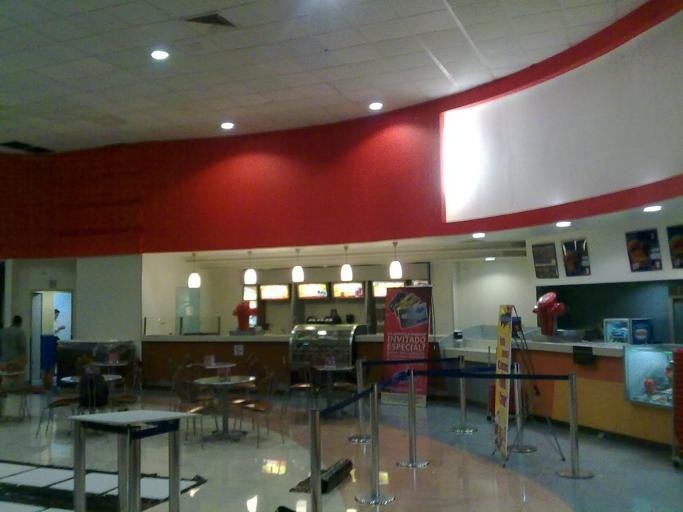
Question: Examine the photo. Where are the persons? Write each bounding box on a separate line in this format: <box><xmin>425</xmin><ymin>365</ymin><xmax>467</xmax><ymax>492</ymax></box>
<box><xmin>0</xmin><ymin>315</ymin><xmax>27</xmax><ymax>361</ymax></box>
<box><xmin>54</xmin><ymin>309</ymin><xmax>65</xmax><ymax>376</ymax></box>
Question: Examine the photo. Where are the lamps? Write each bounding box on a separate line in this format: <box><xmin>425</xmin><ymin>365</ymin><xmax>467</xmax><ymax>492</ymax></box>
<box><xmin>187</xmin><ymin>241</ymin><xmax>403</xmax><ymax>289</ymax></box>
